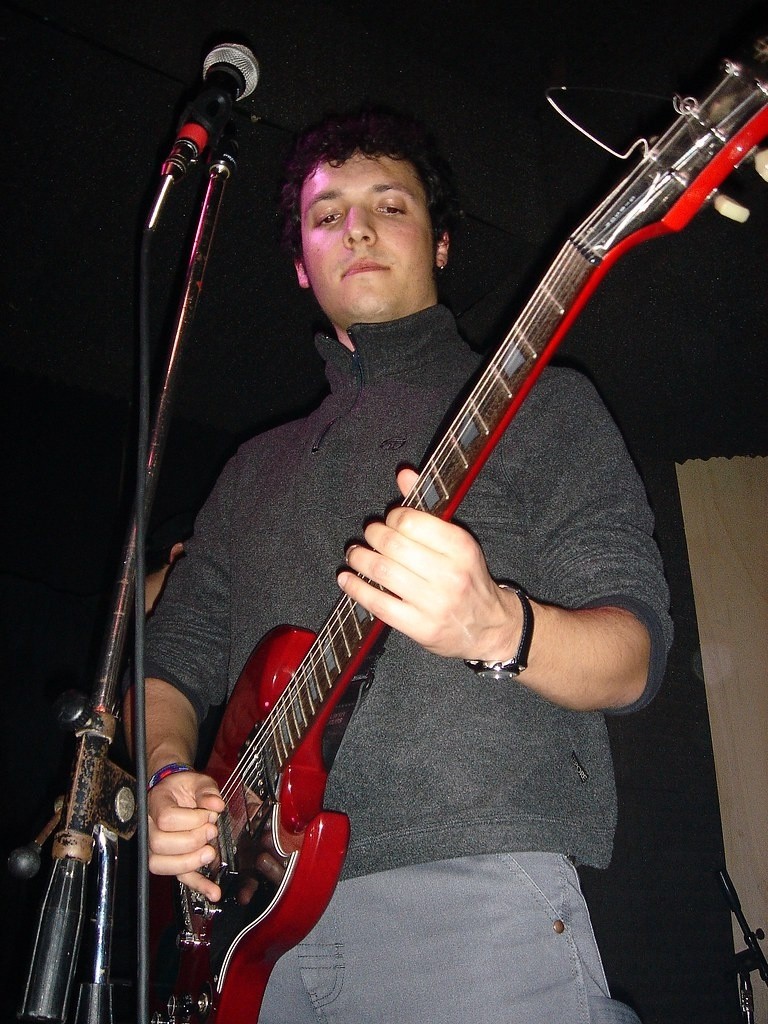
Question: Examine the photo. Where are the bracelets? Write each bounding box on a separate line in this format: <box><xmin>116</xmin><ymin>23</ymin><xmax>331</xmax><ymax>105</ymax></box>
<box><xmin>146</xmin><ymin>762</ymin><xmax>195</xmax><ymax>794</ymax></box>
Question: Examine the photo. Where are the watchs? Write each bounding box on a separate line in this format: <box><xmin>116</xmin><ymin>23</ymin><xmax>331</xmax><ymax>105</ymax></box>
<box><xmin>464</xmin><ymin>579</ymin><xmax>534</xmax><ymax>680</ymax></box>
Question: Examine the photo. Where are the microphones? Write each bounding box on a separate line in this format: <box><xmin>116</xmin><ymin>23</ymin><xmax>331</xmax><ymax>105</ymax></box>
<box><xmin>161</xmin><ymin>42</ymin><xmax>260</xmax><ymax>185</ymax></box>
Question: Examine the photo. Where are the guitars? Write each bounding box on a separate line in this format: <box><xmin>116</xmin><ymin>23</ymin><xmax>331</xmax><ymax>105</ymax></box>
<box><xmin>146</xmin><ymin>32</ymin><xmax>767</xmax><ymax>1024</ymax></box>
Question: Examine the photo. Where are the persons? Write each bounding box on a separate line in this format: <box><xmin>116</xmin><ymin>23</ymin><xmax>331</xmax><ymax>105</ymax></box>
<box><xmin>119</xmin><ymin>112</ymin><xmax>674</xmax><ymax>1023</ymax></box>
